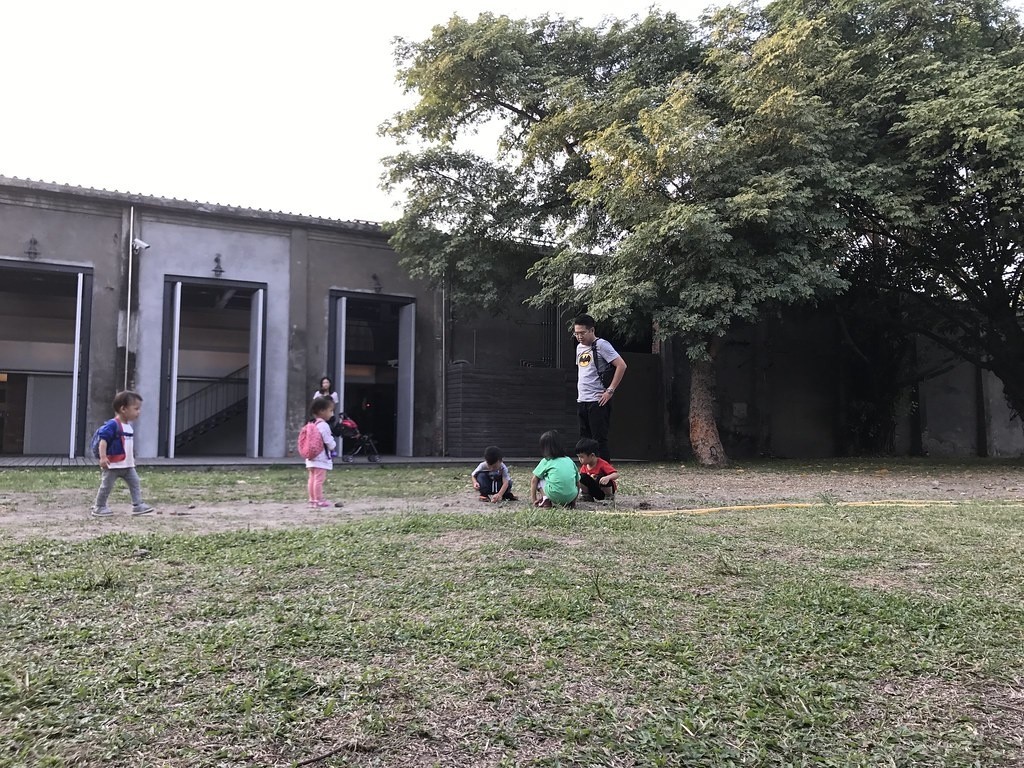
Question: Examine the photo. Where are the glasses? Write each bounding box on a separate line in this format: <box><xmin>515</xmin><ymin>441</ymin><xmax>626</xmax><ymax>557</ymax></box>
<box><xmin>573</xmin><ymin>328</ymin><xmax>592</xmax><ymax>336</ymax></box>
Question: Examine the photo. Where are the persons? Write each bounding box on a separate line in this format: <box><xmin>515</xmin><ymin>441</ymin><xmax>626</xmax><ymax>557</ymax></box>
<box><xmin>575</xmin><ymin>439</ymin><xmax>620</xmax><ymax>506</ymax></box>
<box><xmin>573</xmin><ymin>314</ymin><xmax>627</xmax><ymax>463</ymax></box>
<box><xmin>313</xmin><ymin>377</ymin><xmax>338</xmax><ymax>455</ymax></box>
<box><xmin>472</xmin><ymin>446</ymin><xmax>518</xmax><ymax>502</ymax></box>
<box><xmin>306</xmin><ymin>395</ymin><xmax>336</xmax><ymax>507</ymax></box>
<box><xmin>92</xmin><ymin>391</ymin><xmax>153</xmax><ymax>517</ymax></box>
<box><xmin>531</xmin><ymin>431</ymin><xmax>581</xmax><ymax>509</ymax></box>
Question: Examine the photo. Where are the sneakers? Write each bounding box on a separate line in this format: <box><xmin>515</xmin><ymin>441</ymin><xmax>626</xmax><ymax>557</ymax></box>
<box><xmin>91</xmin><ymin>505</ymin><xmax>114</xmax><ymax>517</ymax></box>
<box><xmin>309</xmin><ymin>498</ymin><xmax>331</xmax><ymax>509</ymax></box>
<box><xmin>132</xmin><ymin>502</ymin><xmax>154</xmax><ymax>515</ymax></box>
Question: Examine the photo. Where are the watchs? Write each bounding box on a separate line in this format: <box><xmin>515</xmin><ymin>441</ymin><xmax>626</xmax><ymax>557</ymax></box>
<box><xmin>607</xmin><ymin>388</ymin><xmax>614</xmax><ymax>394</ymax></box>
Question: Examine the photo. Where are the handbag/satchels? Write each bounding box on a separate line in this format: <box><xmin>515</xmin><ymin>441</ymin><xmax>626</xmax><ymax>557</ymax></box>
<box><xmin>591</xmin><ymin>339</ymin><xmax>624</xmax><ymax>394</ymax></box>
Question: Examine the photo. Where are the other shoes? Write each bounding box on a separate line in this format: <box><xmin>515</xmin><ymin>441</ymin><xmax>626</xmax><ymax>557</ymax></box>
<box><xmin>562</xmin><ymin>500</ymin><xmax>576</xmax><ymax>509</ymax></box>
<box><xmin>534</xmin><ymin>497</ymin><xmax>552</xmax><ymax>508</ymax></box>
<box><xmin>479</xmin><ymin>495</ymin><xmax>491</xmax><ymax>502</ymax></box>
<box><xmin>503</xmin><ymin>493</ymin><xmax>517</xmax><ymax>501</ymax></box>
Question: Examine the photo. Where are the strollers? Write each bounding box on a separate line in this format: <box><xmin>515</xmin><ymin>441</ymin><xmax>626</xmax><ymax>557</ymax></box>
<box><xmin>335</xmin><ymin>413</ymin><xmax>383</xmax><ymax>463</ymax></box>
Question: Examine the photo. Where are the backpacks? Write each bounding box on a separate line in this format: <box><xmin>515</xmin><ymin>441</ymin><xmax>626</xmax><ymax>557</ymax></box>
<box><xmin>91</xmin><ymin>419</ymin><xmax>119</xmax><ymax>459</ymax></box>
<box><xmin>298</xmin><ymin>418</ymin><xmax>325</xmax><ymax>460</ymax></box>
